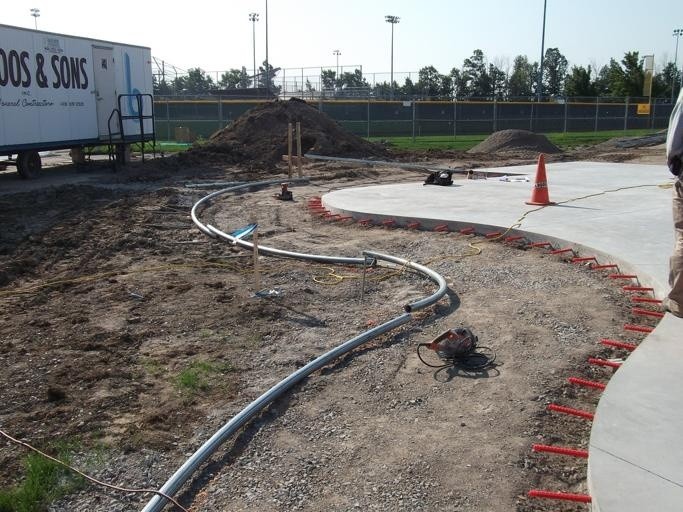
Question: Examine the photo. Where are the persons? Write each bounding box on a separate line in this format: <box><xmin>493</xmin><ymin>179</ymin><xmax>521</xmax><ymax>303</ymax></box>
<box><xmin>660</xmin><ymin>87</ymin><xmax>682</xmax><ymax>318</ymax></box>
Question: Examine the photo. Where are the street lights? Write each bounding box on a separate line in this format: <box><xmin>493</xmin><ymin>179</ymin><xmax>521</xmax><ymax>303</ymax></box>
<box><xmin>670</xmin><ymin>29</ymin><xmax>683</xmax><ymax>104</ymax></box>
<box><xmin>30</xmin><ymin>8</ymin><xmax>42</xmax><ymax>30</ymax></box>
<box><xmin>332</xmin><ymin>49</ymin><xmax>342</xmax><ymax>91</ymax></box>
<box><xmin>384</xmin><ymin>15</ymin><xmax>401</xmax><ymax>87</ymax></box>
<box><xmin>248</xmin><ymin>12</ymin><xmax>259</xmax><ymax>88</ymax></box>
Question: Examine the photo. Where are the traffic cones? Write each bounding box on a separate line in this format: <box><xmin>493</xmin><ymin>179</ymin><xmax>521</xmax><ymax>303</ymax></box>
<box><xmin>524</xmin><ymin>152</ymin><xmax>556</xmax><ymax>206</ymax></box>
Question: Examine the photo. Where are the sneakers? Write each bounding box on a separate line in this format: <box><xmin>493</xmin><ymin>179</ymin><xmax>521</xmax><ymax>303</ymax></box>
<box><xmin>663</xmin><ymin>297</ymin><xmax>683</xmax><ymax>319</ymax></box>
